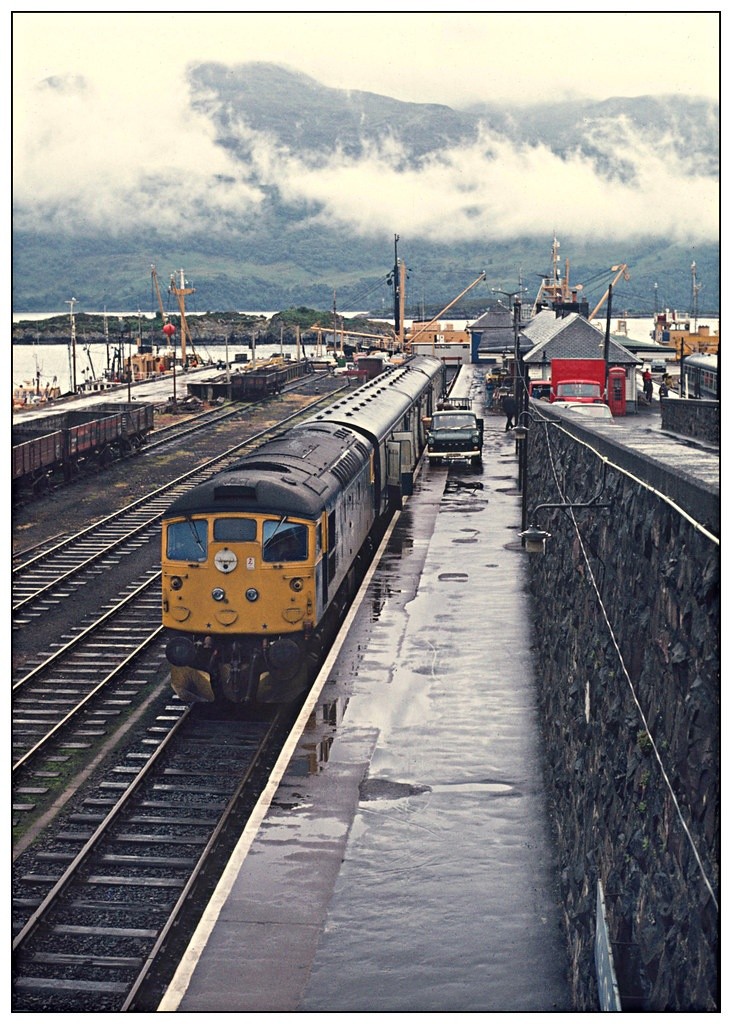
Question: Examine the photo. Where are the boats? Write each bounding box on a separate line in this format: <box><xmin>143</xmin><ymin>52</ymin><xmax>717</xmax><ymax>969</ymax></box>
<box><xmin>13</xmin><ymin>351</ymin><xmax>61</xmax><ymax>408</ymax></box>
<box><xmin>79</xmin><ymin>368</ymin><xmax>123</xmax><ymax>397</ymax></box>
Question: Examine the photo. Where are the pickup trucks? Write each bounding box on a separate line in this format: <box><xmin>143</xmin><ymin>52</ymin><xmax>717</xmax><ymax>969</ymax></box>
<box><xmin>426</xmin><ymin>411</ymin><xmax>485</xmax><ymax>469</ymax></box>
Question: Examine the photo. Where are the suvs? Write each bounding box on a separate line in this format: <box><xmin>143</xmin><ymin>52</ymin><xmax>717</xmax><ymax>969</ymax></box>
<box><xmin>650</xmin><ymin>359</ymin><xmax>666</xmax><ymax>372</ymax></box>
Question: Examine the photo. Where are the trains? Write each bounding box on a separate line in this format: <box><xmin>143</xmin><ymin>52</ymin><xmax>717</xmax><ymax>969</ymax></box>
<box><xmin>682</xmin><ymin>352</ymin><xmax>718</xmax><ymax>400</ymax></box>
<box><xmin>162</xmin><ymin>352</ymin><xmax>448</xmax><ymax>714</ymax></box>
<box><xmin>13</xmin><ymin>402</ymin><xmax>154</xmax><ymax>498</ymax></box>
<box><xmin>232</xmin><ymin>368</ymin><xmax>287</xmax><ymax>400</ymax></box>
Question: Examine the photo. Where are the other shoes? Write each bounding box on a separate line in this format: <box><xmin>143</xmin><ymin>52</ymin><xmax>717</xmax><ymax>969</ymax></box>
<box><xmin>647</xmin><ymin>402</ymin><xmax>650</xmax><ymax>405</ymax></box>
<box><xmin>645</xmin><ymin>398</ymin><xmax>649</xmax><ymax>401</ymax></box>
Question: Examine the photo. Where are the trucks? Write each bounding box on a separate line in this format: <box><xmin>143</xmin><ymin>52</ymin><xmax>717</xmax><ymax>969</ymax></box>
<box><xmin>528</xmin><ymin>358</ymin><xmax>627</xmax><ymax>419</ymax></box>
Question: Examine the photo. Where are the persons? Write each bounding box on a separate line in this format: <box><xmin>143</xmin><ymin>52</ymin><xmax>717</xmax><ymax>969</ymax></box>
<box><xmin>643</xmin><ymin>369</ymin><xmax>651</xmax><ymax>393</ymax></box>
<box><xmin>646</xmin><ymin>378</ymin><xmax>654</xmax><ymax>403</ymax></box>
<box><xmin>666</xmin><ymin>376</ymin><xmax>674</xmax><ymax>389</ymax></box>
<box><xmin>485</xmin><ymin>378</ymin><xmax>496</xmax><ymax>409</ymax></box>
<box><xmin>659</xmin><ymin>381</ymin><xmax>669</xmax><ymax>413</ymax></box>
<box><xmin>503</xmin><ymin>389</ymin><xmax>517</xmax><ymax>433</ymax></box>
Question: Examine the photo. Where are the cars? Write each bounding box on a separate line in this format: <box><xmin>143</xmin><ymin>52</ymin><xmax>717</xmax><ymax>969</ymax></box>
<box><xmin>553</xmin><ymin>402</ymin><xmax>615</xmax><ymax>423</ymax></box>
<box><xmin>487</xmin><ymin>367</ymin><xmax>512</xmax><ymax>385</ymax></box>
